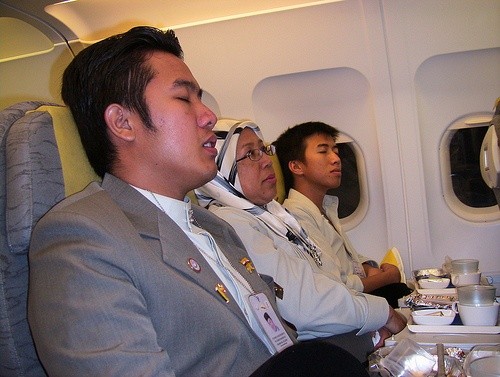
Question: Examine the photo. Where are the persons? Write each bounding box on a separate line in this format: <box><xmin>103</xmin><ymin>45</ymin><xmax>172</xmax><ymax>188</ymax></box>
<box><xmin>27</xmin><ymin>24</ymin><xmax>391</xmax><ymax>377</ymax></box>
<box><xmin>264</xmin><ymin>312</ymin><xmax>280</xmax><ymax>332</ymax></box>
<box><xmin>193</xmin><ymin>118</ymin><xmax>408</xmax><ymax>336</ymax></box>
<box><xmin>275</xmin><ymin>121</ymin><xmax>414</xmax><ymax>309</ymax></box>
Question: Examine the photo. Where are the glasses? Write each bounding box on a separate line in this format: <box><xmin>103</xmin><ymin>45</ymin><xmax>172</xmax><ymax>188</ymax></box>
<box><xmin>236</xmin><ymin>145</ymin><xmax>275</xmax><ymax>162</ymax></box>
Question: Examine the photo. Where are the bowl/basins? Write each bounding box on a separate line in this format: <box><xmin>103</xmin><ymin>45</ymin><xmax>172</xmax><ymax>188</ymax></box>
<box><xmin>462</xmin><ymin>344</ymin><xmax>500</xmax><ymax>377</ymax></box>
<box><xmin>418</xmin><ymin>278</ymin><xmax>450</xmax><ymax>289</ymax></box>
<box><xmin>458</xmin><ymin>303</ymin><xmax>500</xmax><ymax>326</ymax></box>
<box><xmin>458</xmin><ymin>285</ymin><xmax>497</xmax><ymax>306</ymax></box>
<box><xmin>412</xmin><ymin>268</ymin><xmax>450</xmax><ymax>281</ymax></box>
<box><xmin>380</xmin><ymin>339</ymin><xmax>437</xmax><ymax>377</ymax></box>
<box><xmin>450</xmin><ymin>259</ymin><xmax>480</xmax><ymax>272</ymax></box>
<box><xmin>412</xmin><ymin>309</ymin><xmax>457</xmax><ymax>325</ymax></box>
<box><xmin>451</xmin><ymin>273</ymin><xmax>481</xmax><ymax>288</ymax></box>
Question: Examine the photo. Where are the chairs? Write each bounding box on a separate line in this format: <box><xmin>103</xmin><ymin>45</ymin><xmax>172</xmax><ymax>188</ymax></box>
<box><xmin>0</xmin><ymin>100</ymin><xmax>105</xmax><ymax>377</ymax></box>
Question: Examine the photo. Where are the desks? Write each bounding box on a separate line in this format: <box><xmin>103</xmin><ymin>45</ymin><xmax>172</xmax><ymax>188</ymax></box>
<box><xmin>383</xmin><ymin>278</ymin><xmax>500</xmax><ymax>350</ymax></box>
